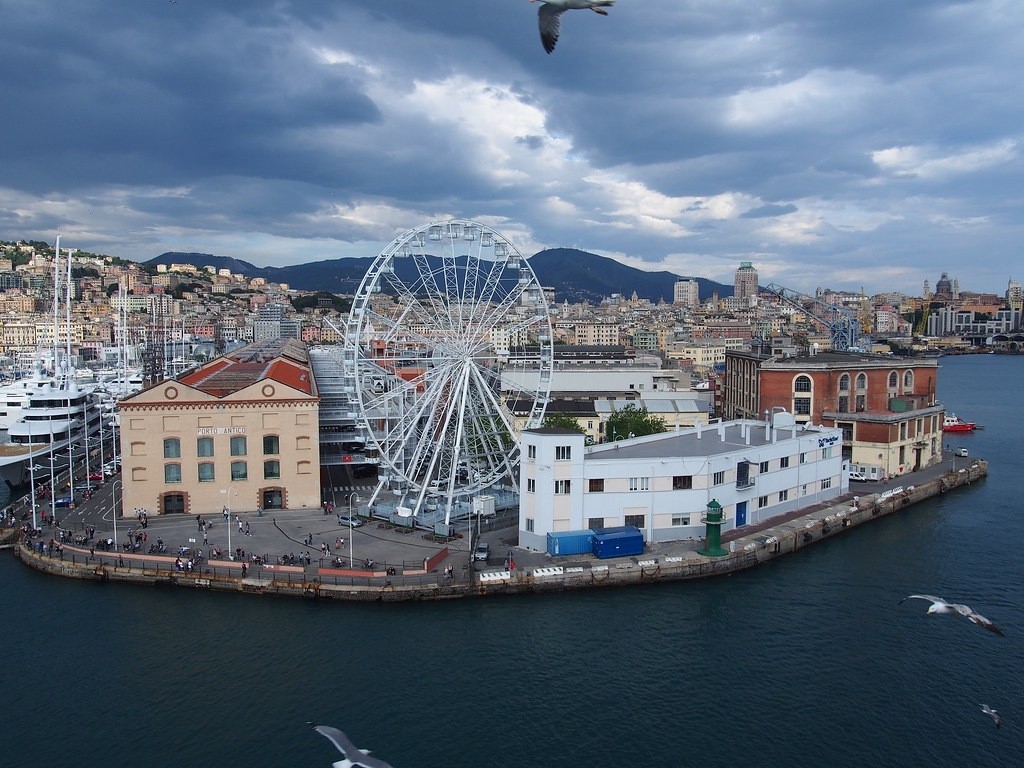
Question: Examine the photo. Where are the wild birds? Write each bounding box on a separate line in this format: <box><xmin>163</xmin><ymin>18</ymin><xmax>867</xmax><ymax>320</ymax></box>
<box><xmin>306</xmin><ymin>722</ymin><xmax>393</xmax><ymax>768</ymax></box>
<box><xmin>978</xmin><ymin>703</ymin><xmax>1001</xmax><ymax>730</ymax></box>
<box><xmin>529</xmin><ymin>0</ymin><xmax>617</xmax><ymax>55</ymax></box>
<box><xmin>897</xmin><ymin>594</ymin><xmax>1008</xmax><ymax>637</ymax></box>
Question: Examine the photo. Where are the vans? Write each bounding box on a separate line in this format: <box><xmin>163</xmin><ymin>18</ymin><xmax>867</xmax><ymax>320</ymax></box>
<box><xmin>955</xmin><ymin>449</ymin><xmax>969</xmax><ymax>457</ymax></box>
<box><xmin>475</xmin><ymin>542</ymin><xmax>490</xmax><ymax>561</ymax></box>
<box><xmin>849</xmin><ymin>472</ymin><xmax>868</xmax><ymax>483</ymax></box>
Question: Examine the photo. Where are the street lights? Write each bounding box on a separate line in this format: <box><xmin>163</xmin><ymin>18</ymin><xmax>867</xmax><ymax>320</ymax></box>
<box><xmin>349</xmin><ymin>492</ymin><xmax>361</xmax><ymax>569</ymax></box>
<box><xmin>455</xmin><ymin>491</ymin><xmax>471</xmax><ymax>580</ymax></box>
<box><xmin>228</xmin><ymin>482</ymin><xmax>237</xmax><ymax>558</ymax></box>
<box><xmin>113</xmin><ymin>480</ymin><xmax>125</xmax><ymax>550</ymax></box>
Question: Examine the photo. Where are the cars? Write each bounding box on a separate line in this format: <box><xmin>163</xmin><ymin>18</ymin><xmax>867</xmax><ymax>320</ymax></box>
<box><xmin>338</xmin><ymin>515</ymin><xmax>362</xmax><ymax>528</ymax></box>
<box><xmin>49</xmin><ymin>498</ymin><xmax>75</xmax><ymax>507</ymax></box>
<box><xmin>75</xmin><ymin>483</ymin><xmax>98</xmax><ymax>491</ymax></box>
<box><xmin>86</xmin><ymin>454</ymin><xmax>121</xmax><ymax>479</ymax></box>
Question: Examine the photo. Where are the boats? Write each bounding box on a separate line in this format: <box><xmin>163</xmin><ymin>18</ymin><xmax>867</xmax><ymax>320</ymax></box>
<box><xmin>943</xmin><ymin>413</ymin><xmax>976</xmax><ymax>431</ymax></box>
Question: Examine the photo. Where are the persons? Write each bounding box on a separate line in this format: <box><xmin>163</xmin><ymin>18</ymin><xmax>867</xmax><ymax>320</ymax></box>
<box><xmin>0</xmin><ymin>448</ymin><xmax>519</xmax><ymax>586</ymax></box>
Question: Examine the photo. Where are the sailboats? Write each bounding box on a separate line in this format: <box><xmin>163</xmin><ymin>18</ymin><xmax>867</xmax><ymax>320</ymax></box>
<box><xmin>0</xmin><ymin>234</ymin><xmax>197</xmax><ymax>492</ymax></box>
